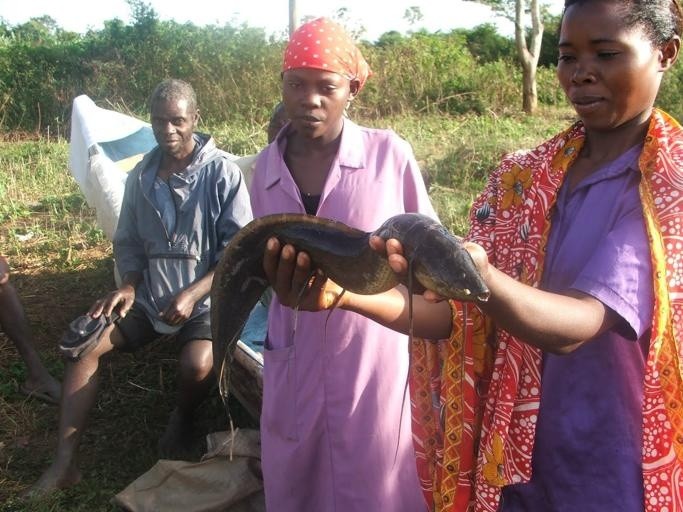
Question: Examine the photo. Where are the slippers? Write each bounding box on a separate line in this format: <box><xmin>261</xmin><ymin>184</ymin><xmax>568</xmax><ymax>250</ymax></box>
<box><xmin>20</xmin><ymin>375</ymin><xmax>62</xmax><ymax>405</ymax></box>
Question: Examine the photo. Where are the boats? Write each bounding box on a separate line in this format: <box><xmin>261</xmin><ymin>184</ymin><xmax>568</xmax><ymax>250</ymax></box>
<box><xmin>72</xmin><ymin>94</ymin><xmax>264</xmax><ymax>425</ymax></box>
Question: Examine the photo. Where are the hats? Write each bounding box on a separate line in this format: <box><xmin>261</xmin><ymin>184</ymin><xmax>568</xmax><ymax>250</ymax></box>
<box><xmin>58</xmin><ymin>305</ymin><xmax>120</xmax><ymax>362</ymax></box>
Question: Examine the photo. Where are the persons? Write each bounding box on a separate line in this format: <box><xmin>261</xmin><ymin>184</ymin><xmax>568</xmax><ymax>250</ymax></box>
<box><xmin>0</xmin><ymin>255</ymin><xmax>62</xmax><ymax>402</ymax></box>
<box><xmin>242</xmin><ymin>16</ymin><xmax>445</xmax><ymax>512</ymax></box>
<box><xmin>260</xmin><ymin>0</ymin><xmax>682</xmax><ymax>511</ymax></box>
<box><xmin>0</xmin><ymin>76</ymin><xmax>255</xmax><ymax>502</ymax></box>
<box><xmin>228</xmin><ymin>99</ymin><xmax>292</xmax><ymax>354</ymax></box>
